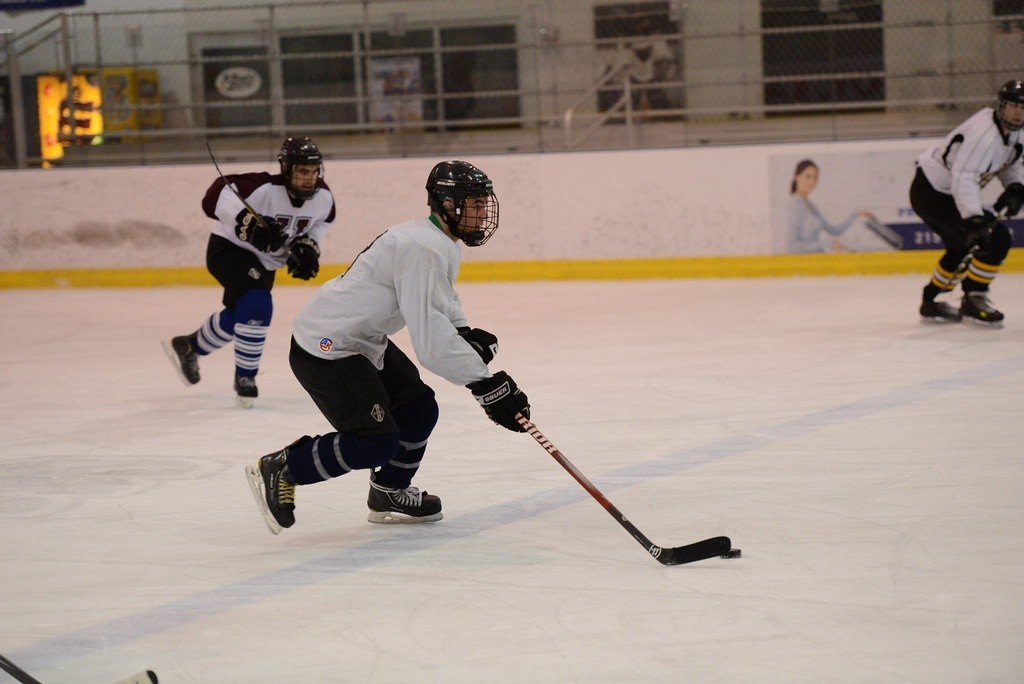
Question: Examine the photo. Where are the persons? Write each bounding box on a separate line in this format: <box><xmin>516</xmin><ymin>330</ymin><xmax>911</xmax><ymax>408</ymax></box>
<box><xmin>171</xmin><ymin>138</ymin><xmax>336</xmax><ymax>398</ymax></box>
<box><xmin>259</xmin><ymin>160</ymin><xmax>530</xmax><ymax>528</ymax></box>
<box><xmin>780</xmin><ymin>160</ymin><xmax>877</xmax><ymax>254</ymax></box>
<box><xmin>909</xmin><ymin>79</ymin><xmax>1024</xmax><ymax>322</ymax></box>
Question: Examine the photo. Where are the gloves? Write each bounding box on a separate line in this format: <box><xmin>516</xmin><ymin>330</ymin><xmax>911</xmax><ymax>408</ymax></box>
<box><xmin>234</xmin><ymin>209</ymin><xmax>287</xmax><ymax>252</ymax></box>
<box><xmin>964</xmin><ymin>215</ymin><xmax>993</xmax><ymax>252</ymax></box>
<box><xmin>456</xmin><ymin>325</ymin><xmax>499</xmax><ymax>365</ymax></box>
<box><xmin>993</xmin><ymin>183</ymin><xmax>1024</xmax><ymax>219</ymax></box>
<box><xmin>465</xmin><ymin>370</ymin><xmax>530</xmax><ymax>433</ymax></box>
<box><xmin>287</xmin><ymin>237</ymin><xmax>319</xmax><ymax>281</ymax></box>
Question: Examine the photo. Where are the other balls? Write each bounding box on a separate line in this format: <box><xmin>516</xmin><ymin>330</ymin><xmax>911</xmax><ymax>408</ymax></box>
<box><xmin>720</xmin><ymin>549</ymin><xmax>742</xmax><ymax>559</ymax></box>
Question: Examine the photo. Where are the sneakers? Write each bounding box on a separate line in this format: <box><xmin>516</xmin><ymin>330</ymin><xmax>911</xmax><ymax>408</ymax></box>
<box><xmin>919</xmin><ymin>288</ymin><xmax>963</xmax><ymax>326</ymax></box>
<box><xmin>233</xmin><ymin>373</ymin><xmax>259</xmax><ymax>408</ymax></box>
<box><xmin>246</xmin><ymin>434</ymin><xmax>311</xmax><ymax>535</ymax></box>
<box><xmin>161</xmin><ymin>331</ymin><xmax>200</xmax><ymax>388</ymax></box>
<box><xmin>960</xmin><ymin>287</ymin><xmax>1004</xmax><ymax>328</ymax></box>
<box><xmin>367</xmin><ymin>466</ymin><xmax>443</xmax><ymax>523</ymax></box>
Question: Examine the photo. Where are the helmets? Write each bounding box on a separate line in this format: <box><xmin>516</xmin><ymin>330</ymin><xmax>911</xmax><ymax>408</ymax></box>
<box><xmin>426</xmin><ymin>160</ymin><xmax>500</xmax><ymax>247</ymax></box>
<box><xmin>278</xmin><ymin>136</ymin><xmax>321</xmax><ymax>198</ymax></box>
<box><xmin>994</xmin><ymin>78</ymin><xmax>1024</xmax><ymax>131</ymax></box>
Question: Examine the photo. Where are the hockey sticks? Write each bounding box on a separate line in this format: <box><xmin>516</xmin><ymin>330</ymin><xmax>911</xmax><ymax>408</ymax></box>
<box><xmin>0</xmin><ymin>654</ymin><xmax>160</xmax><ymax>684</ymax></box>
<box><xmin>942</xmin><ymin>205</ymin><xmax>1008</xmax><ymax>292</ymax></box>
<box><xmin>204</xmin><ymin>140</ymin><xmax>300</xmax><ymax>267</ymax></box>
<box><xmin>516</xmin><ymin>413</ymin><xmax>732</xmax><ymax>567</ymax></box>
